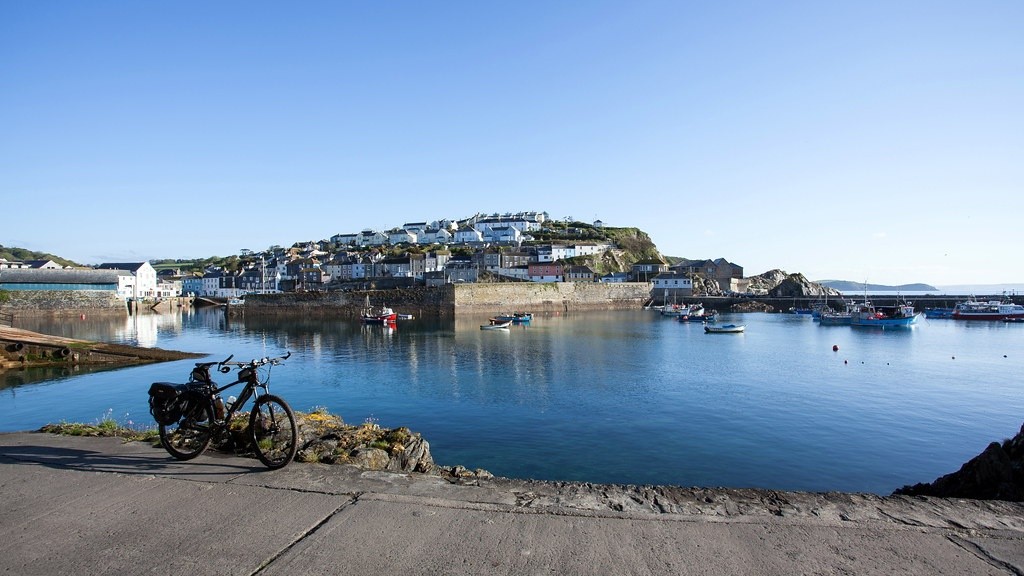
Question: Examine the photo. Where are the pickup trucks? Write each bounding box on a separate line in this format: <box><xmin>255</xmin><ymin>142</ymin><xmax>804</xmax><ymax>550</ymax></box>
<box><xmin>747</xmin><ymin>284</ymin><xmax>769</xmax><ymax>298</ymax></box>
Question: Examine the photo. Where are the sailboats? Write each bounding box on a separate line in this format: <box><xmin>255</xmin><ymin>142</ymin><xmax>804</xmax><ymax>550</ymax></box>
<box><xmin>793</xmin><ymin>287</ymin><xmax>852</xmax><ymax>326</ymax></box>
<box><xmin>359</xmin><ymin>295</ymin><xmax>388</xmax><ymax>324</ymax></box>
<box><xmin>851</xmin><ymin>280</ymin><xmax>916</xmax><ymax>327</ymax></box>
<box><xmin>658</xmin><ymin>291</ymin><xmax>705</xmax><ymax>316</ymax></box>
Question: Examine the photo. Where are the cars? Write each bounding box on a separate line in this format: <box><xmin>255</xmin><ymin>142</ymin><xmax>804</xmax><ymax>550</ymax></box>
<box><xmin>227</xmin><ymin>299</ymin><xmax>245</xmax><ymax>307</ymax></box>
<box><xmin>720</xmin><ymin>289</ymin><xmax>752</xmax><ymax>298</ymax></box>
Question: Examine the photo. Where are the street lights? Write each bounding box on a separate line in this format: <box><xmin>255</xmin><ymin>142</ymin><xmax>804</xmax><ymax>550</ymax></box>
<box><xmin>443</xmin><ymin>266</ymin><xmax>446</xmax><ymax>284</ymax></box>
<box><xmin>497</xmin><ymin>253</ymin><xmax>502</xmax><ymax>282</ymax></box>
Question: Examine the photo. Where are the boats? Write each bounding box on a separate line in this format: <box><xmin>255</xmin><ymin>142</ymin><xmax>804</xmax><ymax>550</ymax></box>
<box><xmin>951</xmin><ymin>297</ymin><xmax>1024</xmax><ymax>319</ymax></box>
<box><xmin>377</xmin><ymin>302</ymin><xmax>397</xmax><ymax>323</ymax></box>
<box><xmin>679</xmin><ymin>313</ymin><xmax>720</xmax><ymax>323</ymax></box>
<box><xmin>398</xmin><ymin>314</ymin><xmax>412</xmax><ymax>321</ymax></box>
<box><xmin>925</xmin><ymin>306</ymin><xmax>953</xmax><ymax>319</ymax></box>
<box><xmin>1002</xmin><ymin>317</ymin><xmax>1024</xmax><ymax>322</ymax></box>
<box><xmin>480</xmin><ymin>312</ymin><xmax>533</xmax><ymax>330</ymax></box>
<box><xmin>704</xmin><ymin>324</ymin><xmax>748</xmax><ymax>333</ymax></box>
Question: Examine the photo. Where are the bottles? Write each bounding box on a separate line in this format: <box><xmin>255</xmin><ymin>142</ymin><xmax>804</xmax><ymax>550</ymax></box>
<box><xmin>215</xmin><ymin>396</ymin><xmax>224</xmax><ymax>420</ymax></box>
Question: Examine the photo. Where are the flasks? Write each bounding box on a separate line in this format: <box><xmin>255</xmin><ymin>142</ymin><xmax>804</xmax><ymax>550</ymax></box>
<box><xmin>223</xmin><ymin>396</ymin><xmax>236</xmax><ymax>413</ymax></box>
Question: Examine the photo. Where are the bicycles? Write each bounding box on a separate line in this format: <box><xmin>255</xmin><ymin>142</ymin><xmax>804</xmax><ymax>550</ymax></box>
<box><xmin>159</xmin><ymin>350</ymin><xmax>302</xmax><ymax>469</ymax></box>
